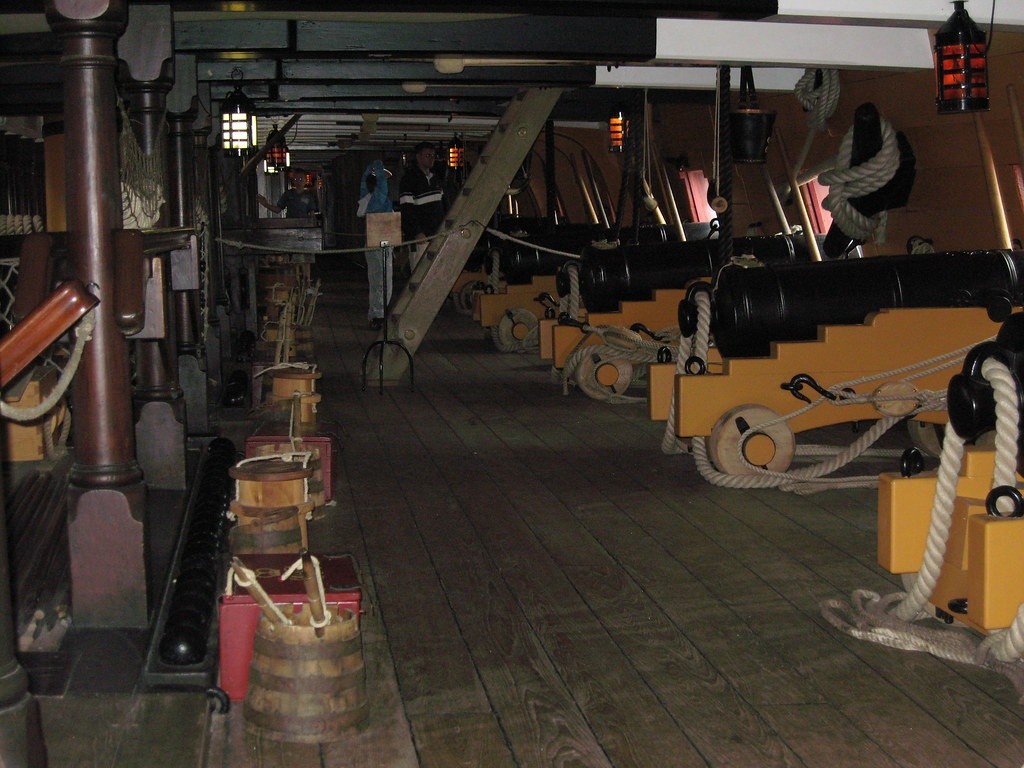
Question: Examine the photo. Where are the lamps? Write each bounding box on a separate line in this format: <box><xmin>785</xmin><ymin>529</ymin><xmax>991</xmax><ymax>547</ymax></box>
<box><xmin>609</xmin><ymin>101</ymin><xmax>632</xmax><ymax>153</ymax></box>
<box><xmin>263</xmin><ymin>124</ymin><xmax>291</xmax><ymax>174</ymax></box>
<box><xmin>447</xmin><ymin>133</ymin><xmax>464</xmax><ymax>170</ymax></box>
<box><xmin>301</xmin><ymin>171</ymin><xmax>313</xmax><ymax>187</ymax></box>
<box><xmin>220</xmin><ymin>68</ymin><xmax>258</xmax><ymax>158</ymax></box>
<box><xmin>933</xmin><ymin>1</ymin><xmax>994</xmax><ymax>115</ymax></box>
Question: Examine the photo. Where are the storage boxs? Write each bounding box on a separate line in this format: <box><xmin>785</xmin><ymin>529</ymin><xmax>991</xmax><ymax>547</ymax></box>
<box><xmin>215</xmin><ymin>551</ymin><xmax>374</xmax><ymax>705</ymax></box>
<box><xmin>245</xmin><ymin>416</ymin><xmax>353</xmax><ymax>505</ymax></box>
<box><xmin>252</xmin><ymin>349</ymin><xmax>318</xmax><ymax>410</ymax></box>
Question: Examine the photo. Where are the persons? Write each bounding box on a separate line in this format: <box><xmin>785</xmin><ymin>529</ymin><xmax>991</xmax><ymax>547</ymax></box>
<box><xmin>399</xmin><ymin>141</ymin><xmax>445</xmax><ymax>274</ymax></box>
<box><xmin>256</xmin><ymin>167</ymin><xmax>317</xmax><ymax>218</ymax></box>
<box><xmin>356</xmin><ymin>171</ymin><xmax>393</xmax><ymax>330</ymax></box>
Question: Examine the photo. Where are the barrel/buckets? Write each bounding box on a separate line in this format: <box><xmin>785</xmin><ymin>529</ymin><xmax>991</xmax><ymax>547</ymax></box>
<box><xmin>243</xmin><ymin>606</ymin><xmax>366</xmax><ymax>741</ymax></box>
<box><xmin>226</xmin><ymin>280</ymin><xmax>324</xmax><ymax>554</ymax></box>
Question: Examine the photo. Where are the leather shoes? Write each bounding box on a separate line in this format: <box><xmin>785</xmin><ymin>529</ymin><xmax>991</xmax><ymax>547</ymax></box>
<box><xmin>370</xmin><ymin>318</ymin><xmax>384</xmax><ymax>330</ymax></box>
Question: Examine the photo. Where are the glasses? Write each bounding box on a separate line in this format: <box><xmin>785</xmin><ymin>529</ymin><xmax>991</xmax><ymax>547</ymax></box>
<box><xmin>293</xmin><ymin>176</ymin><xmax>306</xmax><ymax>182</ymax></box>
<box><xmin>419</xmin><ymin>152</ymin><xmax>435</xmax><ymax>159</ymax></box>
